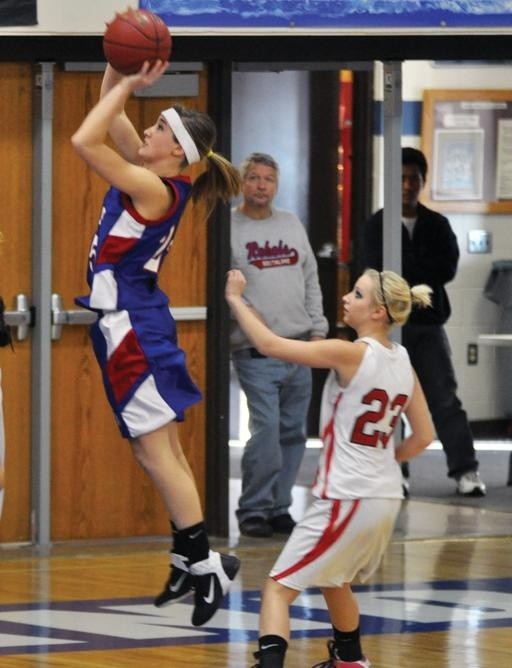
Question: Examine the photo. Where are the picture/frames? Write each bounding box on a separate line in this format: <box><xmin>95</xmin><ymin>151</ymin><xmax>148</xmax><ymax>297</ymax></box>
<box><xmin>414</xmin><ymin>87</ymin><xmax>511</xmax><ymax>216</ymax></box>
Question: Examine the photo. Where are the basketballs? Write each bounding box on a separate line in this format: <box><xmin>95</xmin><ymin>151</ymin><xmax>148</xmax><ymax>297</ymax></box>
<box><xmin>102</xmin><ymin>8</ymin><xmax>172</xmax><ymax>76</ymax></box>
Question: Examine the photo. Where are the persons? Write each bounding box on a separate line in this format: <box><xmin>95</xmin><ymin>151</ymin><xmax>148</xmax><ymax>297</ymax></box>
<box><xmin>365</xmin><ymin>145</ymin><xmax>488</xmax><ymax>499</ymax></box>
<box><xmin>228</xmin><ymin>149</ymin><xmax>327</xmax><ymax>538</ymax></box>
<box><xmin>67</xmin><ymin>6</ymin><xmax>247</xmax><ymax>628</ymax></box>
<box><xmin>224</xmin><ymin>265</ymin><xmax>437</xmax><ymax>667</ymax></box>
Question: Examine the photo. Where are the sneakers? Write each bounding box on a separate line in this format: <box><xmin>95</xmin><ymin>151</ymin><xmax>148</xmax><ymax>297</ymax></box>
<box><xmin>266</xmin><ymin>513</ymin><xmax>297</xmax><ymax>533</ymax></box>
<box><xmin>154</xmin><ymin>547</ymin><xmax>209</xmax><ymax>609</ymax></box>
<box><xmin>457</xmin><ymin>469</ymin><xmax>486</xmax><ymax>497</ymax></box>
<box><xmin>403</xmin><ymin>478</ymin><xmax>412</xmax><ymax>497</ymax></box>
<box><xmin>312</xmin><ymin>640</ymin><xmax>373</xmax><ymax>668</ymax></box>
<box><xmin>239</xmin><ymin>516</ymin><xmax>273</xmax><ymax>537</ymax></box>
<box><xmin>190</xmin><ymin>550</ymin><xmax>240</xmax><ymax>626</ymax></box>
